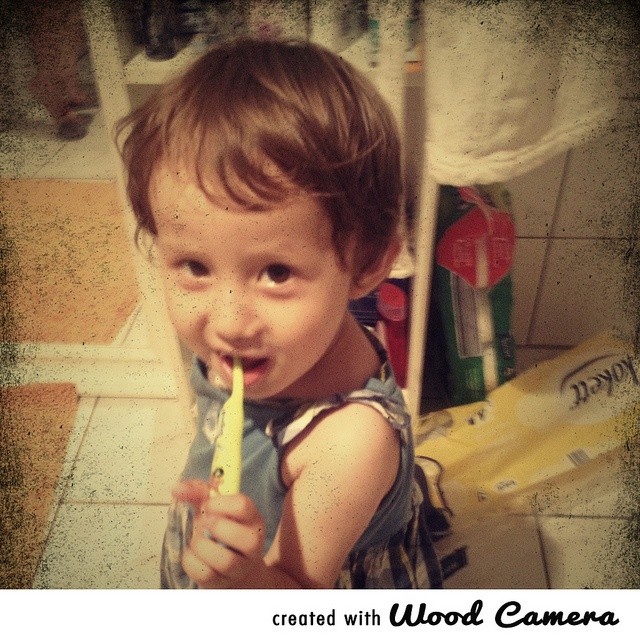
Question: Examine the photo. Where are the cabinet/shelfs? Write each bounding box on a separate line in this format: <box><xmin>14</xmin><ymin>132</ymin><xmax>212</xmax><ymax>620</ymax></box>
<box><xmin>78</xmin><ymin>0</ymin><xmax>440</xmax><ymax>417</ymax></box>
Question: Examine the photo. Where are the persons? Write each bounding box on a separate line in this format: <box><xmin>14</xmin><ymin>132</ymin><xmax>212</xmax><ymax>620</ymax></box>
<box><xmin>106</xmin><ymin>30</ymin><xmax>448</xmax><ymax>589</ymax></box>
